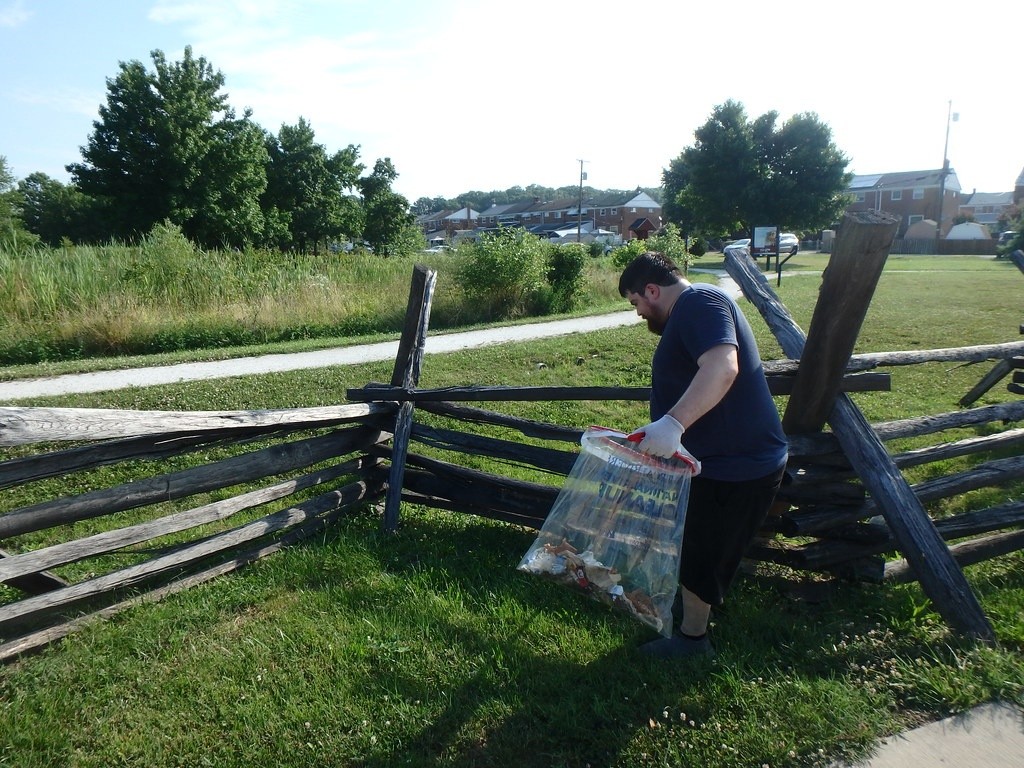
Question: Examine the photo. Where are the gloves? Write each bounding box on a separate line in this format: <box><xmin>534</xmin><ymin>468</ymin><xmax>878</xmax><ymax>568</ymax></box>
<box><xmin>626</xmin><ymin>413</ymin><xmax>686</xmax><ymax>458</ymax></box>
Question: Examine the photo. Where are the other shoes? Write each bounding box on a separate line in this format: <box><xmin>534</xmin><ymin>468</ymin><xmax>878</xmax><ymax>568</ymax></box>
<box><xmin>669</xmin><ymin>600</ymin><xmax>684</xmax><ymax>620</ymax></box>
<box><xmin>636</xmin><ymin>630</ymin><xmax>712</xmax><ymax>658</ymax></box>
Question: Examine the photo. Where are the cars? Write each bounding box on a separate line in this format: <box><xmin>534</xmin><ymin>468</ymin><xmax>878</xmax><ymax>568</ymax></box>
<box><xmin>779</xmin><ymin>234</ymin><xmax>800</xmax><ymax>254</ymax></box>
<box><xmin>722</xmin><ymin>238</ymin><xmax>751</xmax><ymax>256</ymax></box>
<box><xmin>424</xmin><ymin>246</ymin><xmax>459</xmax><ymax>253</ymax></box>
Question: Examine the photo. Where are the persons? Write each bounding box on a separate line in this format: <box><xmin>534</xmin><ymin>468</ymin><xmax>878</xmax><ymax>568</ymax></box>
<box><xmin>618</xmin><ymin>251</ymin><xmax>789</xmax><ymax>659</ymax></box>
